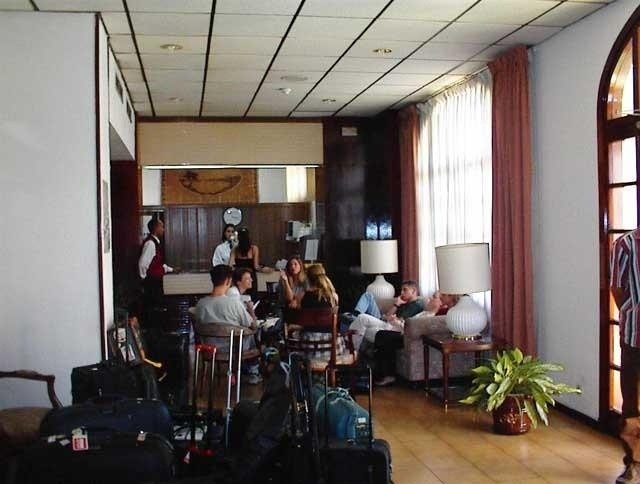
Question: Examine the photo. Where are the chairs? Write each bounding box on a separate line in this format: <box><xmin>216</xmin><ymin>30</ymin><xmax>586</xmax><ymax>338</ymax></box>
<box><xmin>280</xmin><ymin>307</ymin><xmax>357</xmax><ymax>401</ymax></box>
<box><xmin>188</xmin><ymin>313</ymin><xmax>270</xmax><ymax>397</ymax></box>
<box><xmin>265</xmin><ymin>280</ymin><xmax>279</xmax><ymax>300</ymax></box>
<box><xmin>1</xmin><ymin>368</ymin><xmax>62</xmax><ymax>445</ymax></box>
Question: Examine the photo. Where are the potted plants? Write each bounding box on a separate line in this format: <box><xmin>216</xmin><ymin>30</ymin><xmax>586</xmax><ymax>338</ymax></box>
<box><xmin>455</xmin><ymin>346</ymin><xmax>582</xmax><ymax>436</ymax></box>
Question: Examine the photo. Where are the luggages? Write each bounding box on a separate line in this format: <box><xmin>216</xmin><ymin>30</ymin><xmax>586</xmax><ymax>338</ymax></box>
<box><xmin>36</xmin><ymin>310</ymin><xmax>390</xmax><ymax>483</ymax></box>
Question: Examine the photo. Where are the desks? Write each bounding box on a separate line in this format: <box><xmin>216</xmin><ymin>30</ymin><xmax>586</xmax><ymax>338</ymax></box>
<box><xmin>420</xmin><ymin>332</ymin><xmax>508</xmax><ymax>410</ymax></box>
<box><xmin>170</xmin><ymin>316</ymin><xmax>278</xmax><ymax>376</ymax></box>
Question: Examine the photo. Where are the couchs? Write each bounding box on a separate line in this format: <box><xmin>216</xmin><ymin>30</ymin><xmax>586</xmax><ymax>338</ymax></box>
<box><xmin>372</xmin><ymin>296</ymin><xmax>490</xmax><ymax>390</ymax></box>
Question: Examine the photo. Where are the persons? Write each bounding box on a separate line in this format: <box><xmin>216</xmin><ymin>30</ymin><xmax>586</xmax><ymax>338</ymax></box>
<box><xmin>610</xmin><ymin>228</ymin><xmax>640</xmax><ymax>484</ymax></box>
<box><xmin>195</xmin><ymin>255</ymin><xmax>345</xmax><ymax>384</ymax></box>
<box><xmin>342</xmin><ymin>280</ymin><xmax>461</xmax><ymax>386</ymax></box>
<box><xmin>213</xmin><ymin>225</ymin><xmax>271</xmax><ymax>305</ymax></box>
<box><xmin>137</xmin><ymin>218</ymin><xmax>181</xmax><ymax>307</ymax></box>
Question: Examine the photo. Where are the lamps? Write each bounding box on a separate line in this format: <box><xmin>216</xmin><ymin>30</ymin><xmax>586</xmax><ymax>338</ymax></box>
<box><xmin>359</xmin><ymin>238</ymin><xmax>400</xmax><ymax>299</ymax></box>
<box><xmin>435</xmin><ymin>242</ymin><xmax>493</xmax><ymax>341</ymax></box>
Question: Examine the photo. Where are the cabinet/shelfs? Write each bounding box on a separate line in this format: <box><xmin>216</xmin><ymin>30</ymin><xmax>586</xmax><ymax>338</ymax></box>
<box><xmin>224</xmin><ymin>204</ymin><xmax>306</xmax><ymax>266</ymax></box>
<box><xmin>165</xmin><ymin>206</ymin><xmax>224</xmax><ymax>269</ymax></box>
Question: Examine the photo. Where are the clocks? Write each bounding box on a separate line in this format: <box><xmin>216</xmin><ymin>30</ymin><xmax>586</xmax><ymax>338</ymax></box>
<box><xmin>222</xmin><ymin>206</ymin><xmax>244</xmax><ymax>227</ymax></box>
